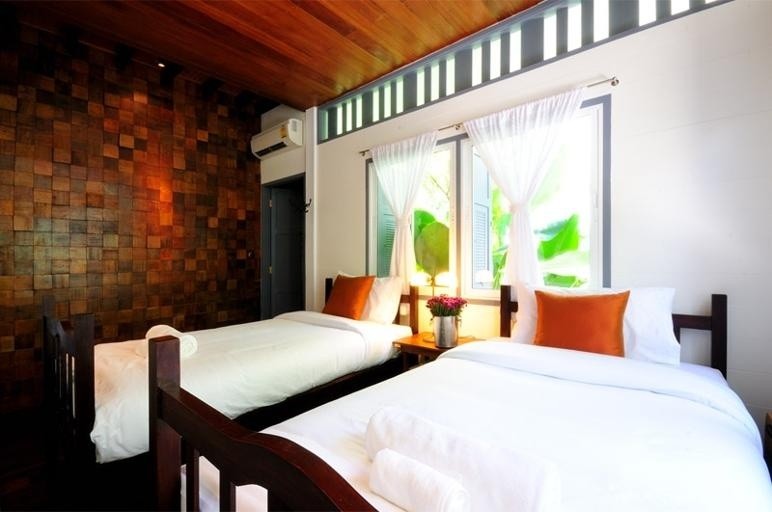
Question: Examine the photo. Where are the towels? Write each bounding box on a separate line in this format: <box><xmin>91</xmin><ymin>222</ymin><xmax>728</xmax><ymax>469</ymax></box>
<box><xmin>145</xmin><ymin>320</ymin><xmax>197</xmax><ymax>361</ymax></box>
<box><xmin>368</xmin><ymin>448</ymin><xmax>470</xmax><ymax>510</ymax></box>
<box><xmin>365</xmin><ymin>407</ymin><xmax>558</xmax><ymax>509</ymax></box>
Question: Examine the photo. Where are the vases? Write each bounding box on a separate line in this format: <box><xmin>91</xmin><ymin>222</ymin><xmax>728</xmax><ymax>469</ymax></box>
<box><xmin>433</xmin><ymin>314</ymin><xmax>459</xmax><ymax>350</ymax></box>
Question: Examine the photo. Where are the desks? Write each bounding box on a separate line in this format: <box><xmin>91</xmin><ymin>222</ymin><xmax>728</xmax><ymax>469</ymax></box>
<box><xmin>392</xmin><ymin>331</ymin><xmax>486</xmax><ymax>372</ymax></box>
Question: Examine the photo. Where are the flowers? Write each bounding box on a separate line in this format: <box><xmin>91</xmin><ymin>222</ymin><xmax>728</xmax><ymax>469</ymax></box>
<box><xmin>426</xmin><ymin>292</ymin><xmax>468</xmax><ymax>316</ymax></box>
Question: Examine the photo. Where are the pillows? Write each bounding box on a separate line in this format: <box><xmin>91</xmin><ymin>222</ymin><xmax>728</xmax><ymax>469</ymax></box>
<box><xmin>322</xmin><ymin>273</ymin><xmax>377</xmax><ymax>322</ymax></box>
<box><xmin>530</xmin><ymin>287</ymin><xmax>631</xmax><ymax>358</ymax></box>
<box><xmin>508</xmin><ymin>285</ymin><xmax>683</xmax><ymax>366</ymax></box>
<box><xmin>360</xmin><ymin>277</ymin><xmax>406</xmax><ymax>325</ymax></box>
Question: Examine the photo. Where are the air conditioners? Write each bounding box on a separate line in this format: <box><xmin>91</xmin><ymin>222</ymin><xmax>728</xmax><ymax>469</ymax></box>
<box><xmin>248</xmin><ymin>117</ymin><xmax>303</xmax><ymax>161</ymax></box>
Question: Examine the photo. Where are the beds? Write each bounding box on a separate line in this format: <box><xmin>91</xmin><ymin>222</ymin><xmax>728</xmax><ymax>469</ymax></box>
<box><xmin>145</xmin><ymin>283</ymin><xmax>772</xmax><ymax>510</ymax></box>
<box><xmin>39</xmin><ymin>271</ymin><xmax>423</xmax><ymax>480</ymax></box>
